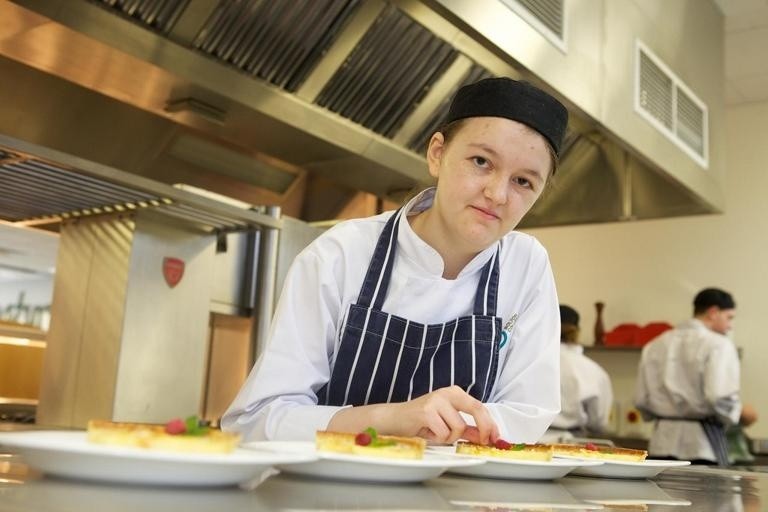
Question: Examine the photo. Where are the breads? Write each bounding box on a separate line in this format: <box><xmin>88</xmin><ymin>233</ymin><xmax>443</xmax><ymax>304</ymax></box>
<box><xmin>549</xmin><ymin>444</ymin><xmax>649</xmax><ymax>463</ymax></box>
<box><xmin>455</xmin><ymin>439</ymin><xmax>554</xmax><ymax>462</ymax></box>
<box><xmin>314</xmin><ymin>429</ymin><xmax>428</xmax><ymax>462</ymax></box>
<box><xmin>86</xmin><ymin>419</ymin><xmax>240</xmax><ymax>456</ymax></box>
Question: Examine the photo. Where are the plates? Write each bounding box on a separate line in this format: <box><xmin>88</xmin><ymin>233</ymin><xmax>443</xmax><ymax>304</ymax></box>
<box><xmin>252</xmin><ymin>474</ymin><xmax>691</xmax><ymax>512</ymax></box>
<box><xmin>0</xmin><ymin>430</ymin><xmax>278</xmax><ymax>487</ymax></box>
<box><xmin>240</xmin><ymin>437</ymin><xmax>487</xmax><ymax>483</ymax></box>
<box><xmin>553</xmin><ymin>457</ymin><xmax>691</xmax><ymax>480</ymax></box>
<box><xmin>426</xmin><ymin>445</ymin><xmax>604</xmax><ymax>483</ymax></box>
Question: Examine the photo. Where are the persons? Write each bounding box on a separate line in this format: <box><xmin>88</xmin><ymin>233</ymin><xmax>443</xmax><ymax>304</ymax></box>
<box><xmin>216</xmin><ymin>76</ymin><xmax>569</xmax><ymax>445</ymax></box>
<box><xmin>541</xmin><ymin>304</ymin><xmax>612</xmax><ymax>445</ymax></box>
<box><xmin>634</xmin><ymin>287</ymin><xmax>759</xmax><ymax>467</ymax></box>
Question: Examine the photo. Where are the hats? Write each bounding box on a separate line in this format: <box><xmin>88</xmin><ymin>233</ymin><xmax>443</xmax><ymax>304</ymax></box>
<box><xmin>444</xmin><ymin>76</ymin><xmax>570</xmax><ymax>159</ymax></box>
<box><xmin>559</xmin><ymin>304</ymin><xmax>580</xmax><ymax>327</ymax></box>
<box><xmin>693</xmin><ymin>288</ymin><xmax>736</xmax><ymax>313</ymax></box>
